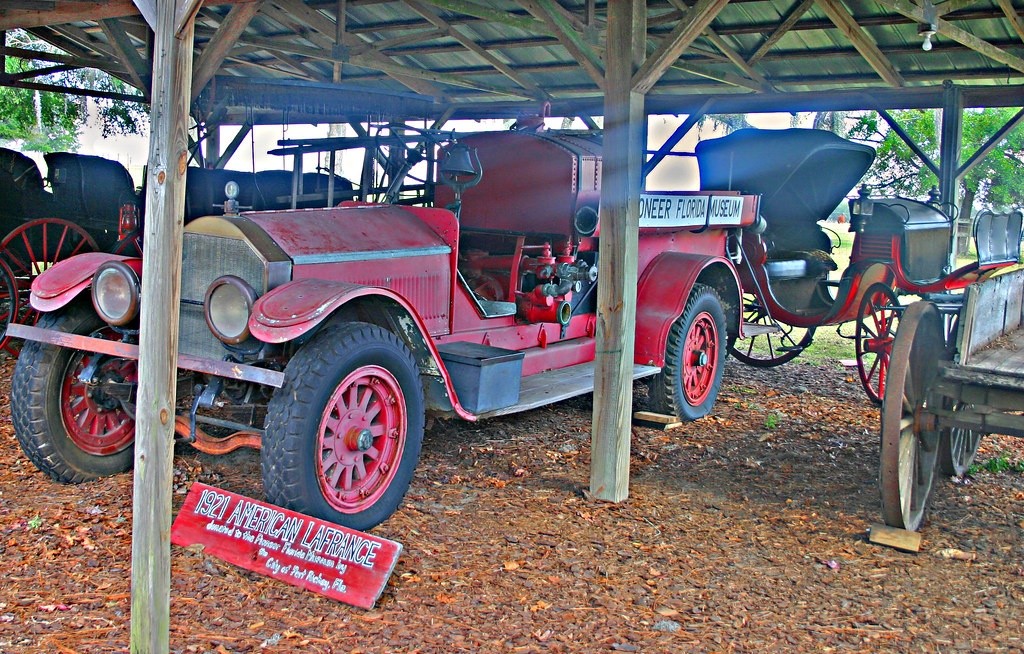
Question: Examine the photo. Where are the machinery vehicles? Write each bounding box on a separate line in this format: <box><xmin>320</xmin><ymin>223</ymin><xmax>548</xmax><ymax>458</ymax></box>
<box><xmin>3</xmin><ymin>100</ymin><xmax>779</xmax><ymax>534</ymax></box>
